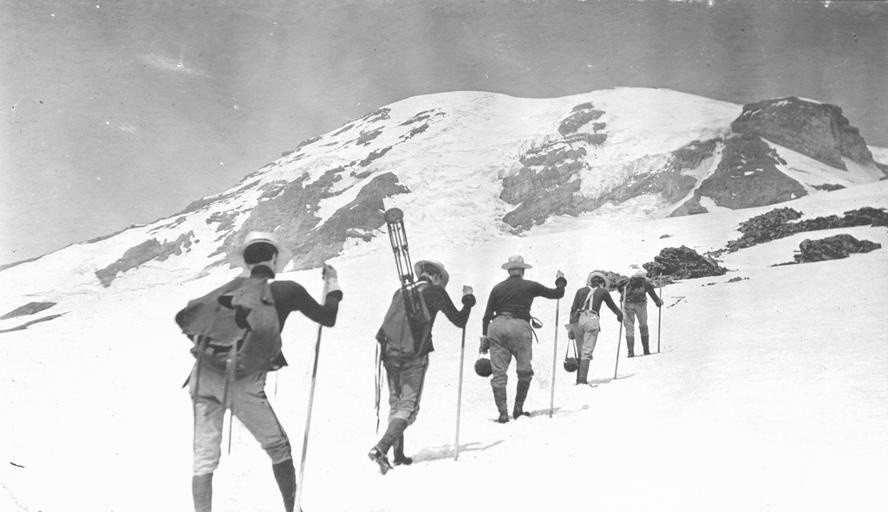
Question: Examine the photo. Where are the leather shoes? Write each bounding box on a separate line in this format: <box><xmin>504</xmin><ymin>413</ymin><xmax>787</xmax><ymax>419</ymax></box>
<box><xmin>512</xmin><ymin>410</ymin><xmax>531</xmax><ymax>418</ymax></box>
<box><xmin>392</xmin><ymin>456</ymin><xmax>414</xmax><ymax>466</ymax></box>
<box><xmin>369</xmin><ymin>448</ymin><xmax>394</xmax><ymax>474</ymax></box>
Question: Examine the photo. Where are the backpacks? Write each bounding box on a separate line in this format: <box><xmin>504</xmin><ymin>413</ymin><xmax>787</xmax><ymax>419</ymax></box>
<box><xmin>175</xmin><ymin>278</ymin><xmax>281</xmax><ymax>378</ymax></box>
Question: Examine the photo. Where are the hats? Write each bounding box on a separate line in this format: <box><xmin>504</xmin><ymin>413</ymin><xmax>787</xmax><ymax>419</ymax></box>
<box><xmin>414</xmin><ymin>260</ymin><xmax>450</xmax><ymax>288</ymax></box>
<box><xmin>586</xmin><ymin>272</ymin><xmax>610</xmax><ymax>290</ymax></box>
<box><xmin>502</xmin><ymin>256</ymin><xmax>531</xmax><ymax>270</ymax></box>
<box><xmin>233</xmin><ymin>230</ymin><xmax>293</xmax><ymax>274</ymax></box>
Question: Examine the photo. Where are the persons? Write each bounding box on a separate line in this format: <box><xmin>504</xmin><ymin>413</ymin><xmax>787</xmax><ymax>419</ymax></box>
<box><xmin>171</xmin><ymin>227</ymin><xmax>345</xmax><ymax>511</ymax></box>
<box><xmin>561</xmin><ymin>272</ymin><xmax>622</xmax><ymax>387</ymax></box>
<box><xmin>365</xmin><ymin>257</ymin><xmax>476</xmax><ymax>475</ymax></box>
<box><xmin>616</xmin><ymin>269</ymin><xmax>665</xmax><ymax>357</ymax></box>
<box><xmin>480</xmin><ymin>254</ymin><xmax>569</xmax><ymax>422</ymax></box>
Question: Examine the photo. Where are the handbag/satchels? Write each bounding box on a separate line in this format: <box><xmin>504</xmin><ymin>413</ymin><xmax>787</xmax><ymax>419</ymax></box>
<box><xmin>578</xmin><ymin>309</ymin><xmax>600</xmax><ymax>334</ymax></box>
